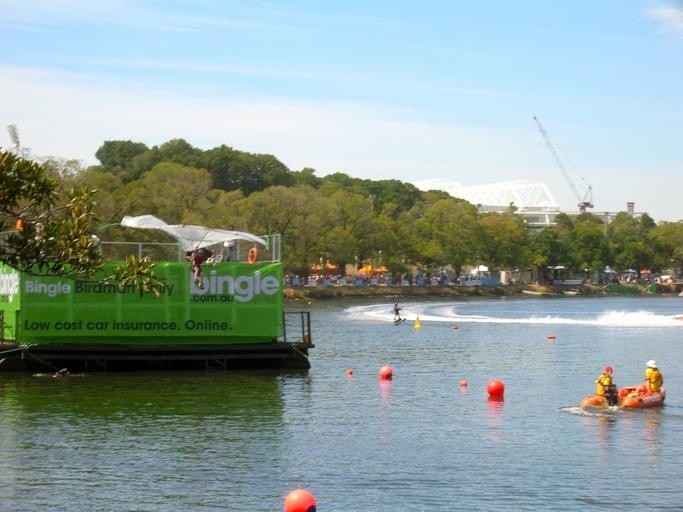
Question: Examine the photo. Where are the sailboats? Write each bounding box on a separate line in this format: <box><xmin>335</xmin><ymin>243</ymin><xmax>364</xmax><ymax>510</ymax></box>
<box><xmin>394</xmin><ymin>316</ymin><xmax>405</xmax><ymax>325</ymax></box>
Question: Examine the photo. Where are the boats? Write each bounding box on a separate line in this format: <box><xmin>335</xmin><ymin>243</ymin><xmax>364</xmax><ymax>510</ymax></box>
<box><xmin>579</xmin><ymin>385</ymin><xmax>665</xmax><ymax>409</ymax></box>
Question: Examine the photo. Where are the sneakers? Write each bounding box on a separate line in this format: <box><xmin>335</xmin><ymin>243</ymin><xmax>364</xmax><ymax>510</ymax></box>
<box><xmin>195</xmin><ymin>279</ymin><xmax>204</xmax><ymax>289</ymax></box>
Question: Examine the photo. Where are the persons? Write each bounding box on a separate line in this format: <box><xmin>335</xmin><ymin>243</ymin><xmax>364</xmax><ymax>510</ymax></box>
<box><xmin>595</xmin><ymin>368</ymin><xmax>612</xmax><ymax>396</ymax></box>
<box><xmin>190</xmin><ymin>249</ymin><xmax>204</xmax><ymax>288</ymax></box>
<box><xmin>15</xmin><ymin>213</ymin><xmax>25</xmax><ymax>230</ymax></box>
<box><xmin>634</xmin><ymin>360</ymin><xmax>662</xmax><ymax>393</ymax></box>
<box><xmin>394</xmin><ymin>303</ymin><xmax>401</xmax><ymax>320</ymax></box>
<box><xmin>89</xmin><ymin>232</ymin><xmax>100</xmax><ymax>242</ymax></box>
<box><xmin>284</xmin><ymin>272</ymin><xmax>471</xmax><ymax>287</ymax></box>
<box><xmin>36</xmin><ymin>214</ymin><xmax>44</xmax><ymax>239</ymax></box>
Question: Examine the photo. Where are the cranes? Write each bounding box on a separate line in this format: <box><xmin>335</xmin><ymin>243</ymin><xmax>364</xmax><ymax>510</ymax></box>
<box><xmin>532</xmin><ymin>115</ymin><xmax>593</xmax><ymax>214</ymax></box>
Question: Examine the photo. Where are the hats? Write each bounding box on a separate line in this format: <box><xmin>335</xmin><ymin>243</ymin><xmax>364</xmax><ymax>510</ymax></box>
<box><xmin>606</xmin><ymin>367</ymin><xmax>612</xmax><ymax>374</ymax></box>
<box><xmin>198</xmin><ymin>250</ymin><xmax>204</xmax><ymax>252</ymax></box>
<box><xmin>646</xmin><ymin>360</ymin><xmax>657</xmax><ymax>368</ymax></box>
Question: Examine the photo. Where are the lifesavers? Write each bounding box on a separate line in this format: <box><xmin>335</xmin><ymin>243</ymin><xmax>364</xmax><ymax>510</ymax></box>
<box><xmin>248</xmin><ymin>248</ymin><xmax>257</xmax><ymax>263</ymax></box>
